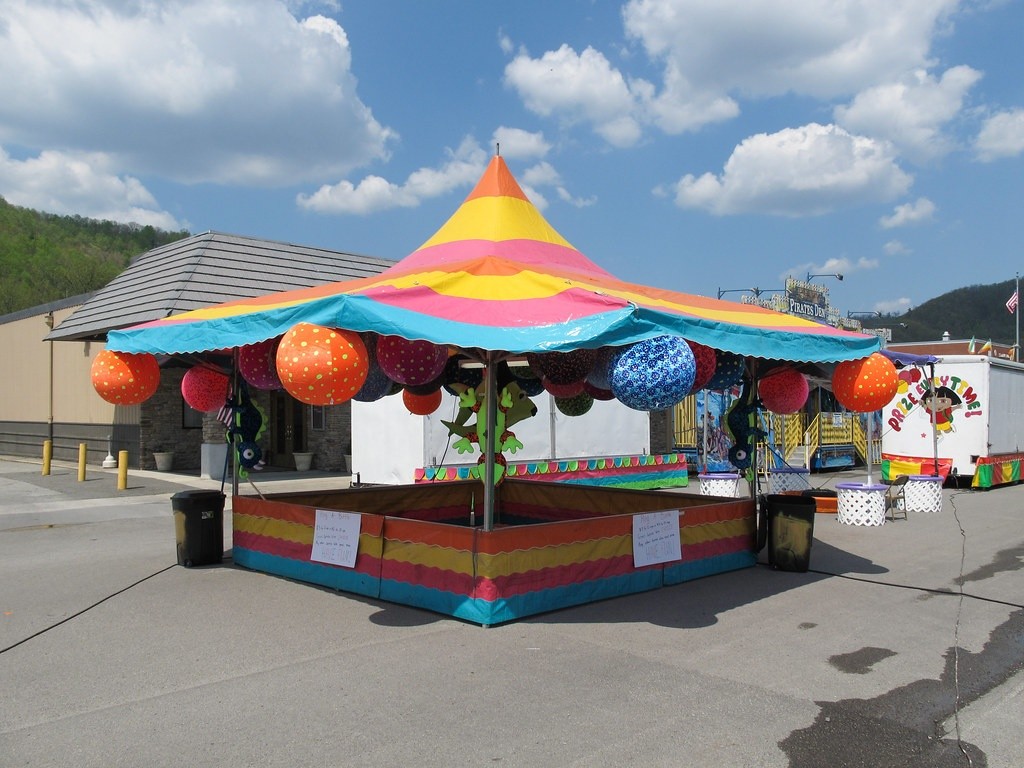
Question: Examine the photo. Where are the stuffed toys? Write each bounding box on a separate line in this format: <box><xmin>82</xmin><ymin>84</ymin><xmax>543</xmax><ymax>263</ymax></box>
<box><xmin>445</xmin><ymin>374</ymin><xmax>538</xmax><ymax>489</ymax></box>
<box><xmin>225</xmin><ymin>370</ymin><xmax>267</xmax><ymax>478</ymax></box>
<box><xmin>718</xmin><ymin>364</ymin><xmax>769</xmax><ymax>481</ymax></box>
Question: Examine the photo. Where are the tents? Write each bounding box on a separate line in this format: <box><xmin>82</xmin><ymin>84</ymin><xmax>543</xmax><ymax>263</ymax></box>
<box><xmin>104</xmin><ymin>141</ymin><xmax>883</xmax><ymax>625</ymax></box>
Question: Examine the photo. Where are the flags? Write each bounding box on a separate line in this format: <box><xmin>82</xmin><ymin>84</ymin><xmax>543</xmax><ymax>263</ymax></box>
<box><xmin>977</xmin><ymin>338</ymin><xmax>991</xmax><ymax>355</ymax></box>
<box><xmin>967</xmin><ymin>336</ymin><xmax>976</xmax><ymax>354</ymax></box>
<box><xmin>1008</xmin><ymin>345</ymin><xmax>1015</xmax><ymax>361</ymax></box>
<box><xmin>1005</xmin><ymin>288</ymin><xmax>1017</xmax><ymax>314</ymax></box>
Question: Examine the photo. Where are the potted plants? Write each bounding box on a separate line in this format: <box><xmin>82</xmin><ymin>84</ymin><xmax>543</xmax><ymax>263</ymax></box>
<box><xmin>152</xmin><ymin>440</ymin><xmax>174</xmax><ymax>471</ymax></box>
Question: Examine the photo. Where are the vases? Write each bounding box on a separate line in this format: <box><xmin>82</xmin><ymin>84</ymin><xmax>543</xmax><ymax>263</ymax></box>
<box><xmin>292</xmin><ymin>452</ymin><xmax>314</xmax><ymax>472</ymax></box>
<box><xmin>343</xmin><ymin>454</ymin><xmax>351</xmax><ymax>473</ymax></box>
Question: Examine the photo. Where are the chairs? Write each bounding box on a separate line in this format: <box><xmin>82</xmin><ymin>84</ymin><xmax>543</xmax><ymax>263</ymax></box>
<box><xmin>884</xmin><ymin>475</ymin><xmax>909</xmax><ymax>523</ymax></box>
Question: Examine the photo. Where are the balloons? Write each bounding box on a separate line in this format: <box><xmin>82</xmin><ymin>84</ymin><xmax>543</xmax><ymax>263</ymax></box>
<box><xmin>91</xmin><ymin>346</ymin><xmax>160</xmax><ymax>406</ymax></box>
<box><xmin>833</xmin><ymin>351</ymin><xmax>899</xmax><ymax>413</ymax></box>
<box><xmin>758</xmin><ymin>364</ymin><xmax>808</xmax><ymax>415</ymax></box>
<box><xmin>275</xmin><ymin>321</ymin><xmax>369</xmax><ymax>405</ymax></box>
<box><xmin>238</xmin><ymin>334</ymin><xmax>285</xmax><ymax>390</ymax></box>
<box><xmin>181</xmin><ymin>363</ymin><xmax>232</xmax><ymax>412</ymax></box>
<box><xmin>352</xmin><ymin>331</ymin><xmax>745</xmax><ymax>418</ymax></box>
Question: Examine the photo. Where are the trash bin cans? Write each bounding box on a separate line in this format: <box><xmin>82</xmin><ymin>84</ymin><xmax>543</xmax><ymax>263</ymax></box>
<box><xmin>169</xmin><ymin>488</ymin><xmax>227</xmax><ymax>569</ymax></box>
<box><xmin>755</xmin><ymin>493</ymin><xmax>818</xmax><ymax>574</ymax></box>
